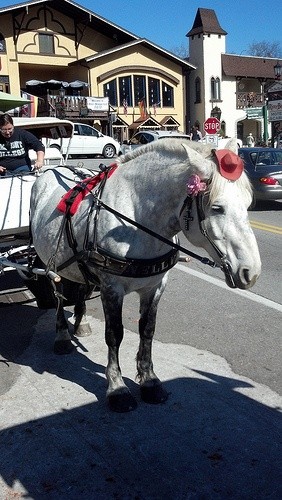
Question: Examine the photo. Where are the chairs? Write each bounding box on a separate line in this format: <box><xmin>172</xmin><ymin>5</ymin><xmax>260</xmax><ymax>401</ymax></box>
<box><xmin>260</xmin><ymin>159</ymin><xmax>274</xmax><ymax>165</ymax></box>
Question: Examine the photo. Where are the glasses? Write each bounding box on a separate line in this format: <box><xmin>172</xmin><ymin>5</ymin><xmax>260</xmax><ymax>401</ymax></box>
<box><xmin>0</xmin><ymin>126</ymin><xmax>14</xmax><ymax>133</ymax></box>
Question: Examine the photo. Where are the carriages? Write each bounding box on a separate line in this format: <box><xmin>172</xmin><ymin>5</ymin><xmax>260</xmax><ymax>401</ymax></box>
<box><xmin>0</xmin><ymin>115</ymin><xmax>264</xmax><ymax>415</ymax></box>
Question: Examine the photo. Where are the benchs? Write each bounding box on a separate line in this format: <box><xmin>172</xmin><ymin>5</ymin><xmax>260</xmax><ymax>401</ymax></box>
<box><xmin>29</xmin><ymin>147</ymin><xmax>65</xmax><ymax>174</ymax></box>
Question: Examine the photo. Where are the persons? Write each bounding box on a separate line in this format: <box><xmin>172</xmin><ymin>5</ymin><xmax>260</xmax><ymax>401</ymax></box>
<box><xmin>190</xmin><ymin>127</ymin><xmax>202</xmax><ymax>142</ymax></box>
<box><xmin>0</xmin><ymin>114</ymin><xmax>45</xmax><ymax>178</ymax></box>
<box><xmin>19</xmin><ymin>106</ymin><xmax>28</xmax><ymax>117</ymax></box>
<box><xmin>246</xmin><ymin>132</ymin><xmax>254</xmax><ymax>148</ymax></box>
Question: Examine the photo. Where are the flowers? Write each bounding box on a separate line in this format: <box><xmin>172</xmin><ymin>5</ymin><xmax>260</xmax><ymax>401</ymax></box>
<box><xmin>186</xmin><ymin>174</ymin><xmax>208</xmax><ymax>196</ymax></box>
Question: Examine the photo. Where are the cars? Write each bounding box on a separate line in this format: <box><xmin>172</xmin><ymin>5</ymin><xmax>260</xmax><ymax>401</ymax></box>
<box><xmin>41</xmin><ymin>123</ymin><xmax>121</xmax><ymax>159</ymax></box>
<box><xmin>237</xmin><ymin>147</ymin><xmax>282</xmax><ymax>211</ymax></box>
<box><xmin>118</xmin><ymin>130</ymin><xmax>192</xmax><ymax>158</ymax></box>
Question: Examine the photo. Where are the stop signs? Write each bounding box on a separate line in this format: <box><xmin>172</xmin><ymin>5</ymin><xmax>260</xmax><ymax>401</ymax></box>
<box><xmin>203</xmin><ymin>117</ymin><xmax>221</xmax><ymax>134</ymax></box>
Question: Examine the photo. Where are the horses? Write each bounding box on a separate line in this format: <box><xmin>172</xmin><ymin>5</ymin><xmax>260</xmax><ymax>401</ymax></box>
<box><xmin>28</xmin><ymin>134</ymin><xmax>265</xmax><ymax>416</ymax></box>
